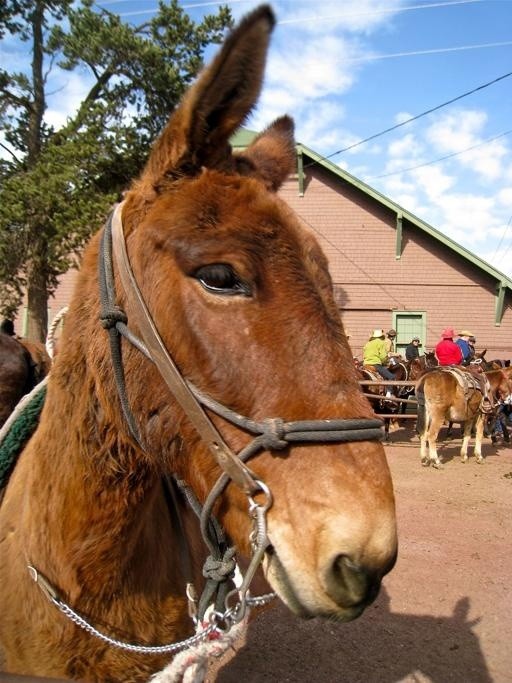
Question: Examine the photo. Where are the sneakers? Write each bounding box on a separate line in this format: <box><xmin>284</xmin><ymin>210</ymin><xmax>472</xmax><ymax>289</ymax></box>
<box><xmin>385</xmin><ymin>395</ymin><xmax>397</xmax><ymax>408</ymax></box>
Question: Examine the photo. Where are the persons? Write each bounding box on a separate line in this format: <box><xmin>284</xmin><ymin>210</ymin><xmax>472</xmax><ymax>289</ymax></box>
<box><xmin>347</xmin><ymin>327</ymin><xmax>505</xmax><ymax>447</ymax></box>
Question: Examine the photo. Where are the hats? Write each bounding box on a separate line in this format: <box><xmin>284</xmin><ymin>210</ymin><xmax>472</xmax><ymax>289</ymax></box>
<box><xmin>443</xmin><ymin>330</ymin><xmax>454</xmax><ymax>339</ymax></box>
<box><xmin>387</xmin><ymin>330</ymin><xmax>397</xmax><ymax>337</ymax></box>
<box><xmin>373</xmin><ymin>330</ymin><xmax>385</xmax><ymax>337</ymax></box>
<box><xmin>470</xmin><ymin>337</ymin><xmax>476</xmax><ymax>341</ymax></box>
<box><xmin>413</xmin><ymin>337</ymin><xmax>419</xmax><ymax>342</ymax></box>
<box><xmin>458</xmin><ymin>331</ymin><xmax>473</xmax><ymax>337</ymax></box>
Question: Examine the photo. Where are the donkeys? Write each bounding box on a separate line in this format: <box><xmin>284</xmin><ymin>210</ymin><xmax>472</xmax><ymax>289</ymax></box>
<box><xmin>0</xmin><ymin>3</ymin><xmax>397</xmax><ymax>682</ymax></box>
<box><xmin>353</xmin><ymin>349</ymin><xmax>512</xmax><ymax>469</ymax></box>
